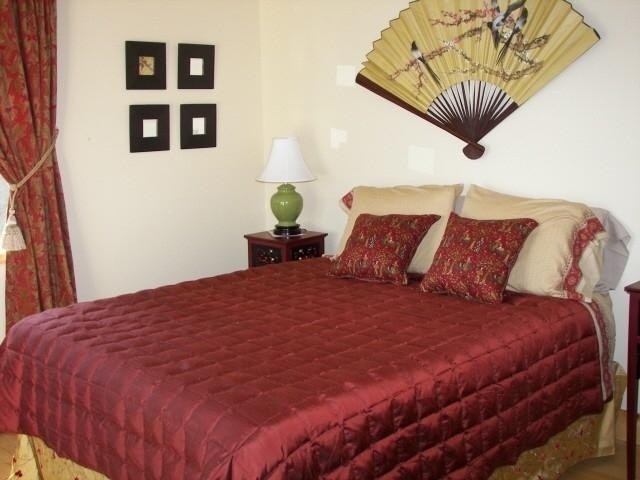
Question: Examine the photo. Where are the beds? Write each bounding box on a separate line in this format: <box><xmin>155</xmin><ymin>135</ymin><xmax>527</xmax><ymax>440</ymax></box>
<box><xmin>1</xmin><ymin>207</ymin><xmax>632</xmax><ymax>479</ymax></box>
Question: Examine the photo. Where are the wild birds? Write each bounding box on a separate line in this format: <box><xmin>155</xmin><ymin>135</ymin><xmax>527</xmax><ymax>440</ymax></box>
<box><xmin>410</xmin><ymin>40</ymin><xmax>443</xmax><ymax>90</ymax></box>
<box><xmin>487</xmin><ymin>0</ymin><xmax>529</xmax><ymax>65</ymax></box>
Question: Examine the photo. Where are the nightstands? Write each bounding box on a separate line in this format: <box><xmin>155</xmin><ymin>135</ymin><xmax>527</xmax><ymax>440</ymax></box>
<box><xmin>244</xmin><ymin>227</ymin><xmax>328</xmax><ymax>269</ymax></box>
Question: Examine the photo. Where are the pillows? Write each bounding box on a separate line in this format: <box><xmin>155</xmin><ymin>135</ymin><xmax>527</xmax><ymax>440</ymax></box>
<box><xmin>325</xmin><ymin>184</ymin><xmax>606</xmax><ymax>302</ymax></box>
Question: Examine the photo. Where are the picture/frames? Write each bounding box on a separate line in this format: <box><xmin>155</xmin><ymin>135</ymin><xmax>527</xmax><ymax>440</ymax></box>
<box><xmin>180</xmin><ymin>104</ymin><xmax>216</xmax><ymax>148</ymax></box>
<box><xmin>178</xmin><ymin>43</ymin><xmax>215</xmax><ymax>89</ymax></box>
<box><xmin>129</xmin><ymin>105</ymin><xmax>169</xmax><ymax>151</ymax></box>
<box><xmin>125</xmin><ymin>41</ymin><xmax>167</xmax><ymax>90</ymax></box>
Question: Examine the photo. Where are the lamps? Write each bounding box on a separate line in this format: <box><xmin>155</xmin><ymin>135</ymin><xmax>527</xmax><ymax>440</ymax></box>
<box><xmin>257</xmin><ymin>138</ymin><xmax>317</xmax><ymax>236</ymax></box>
